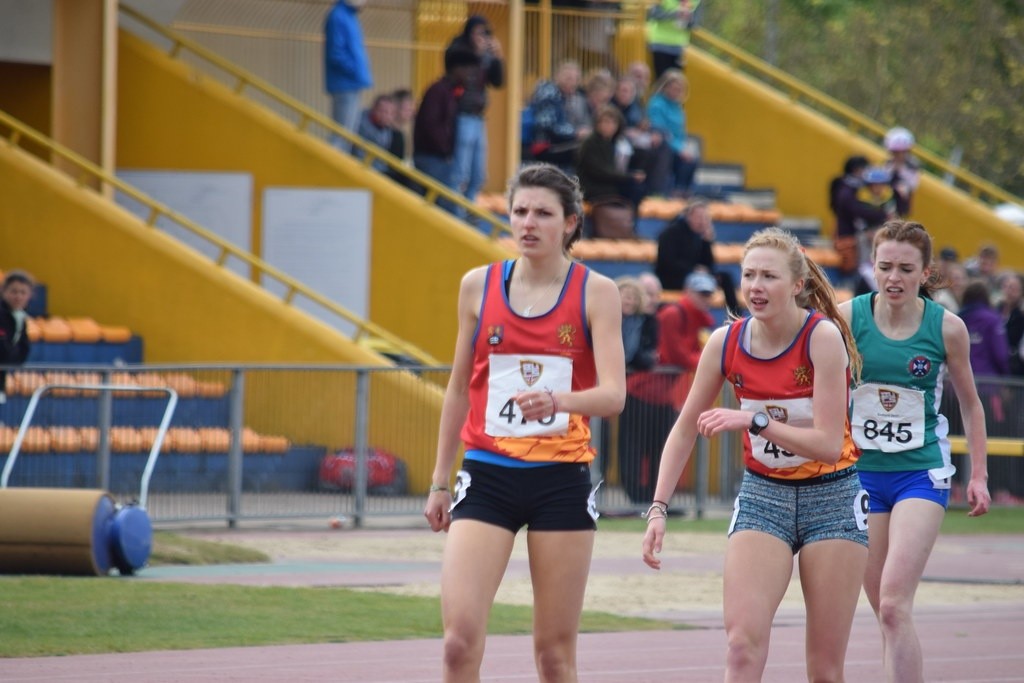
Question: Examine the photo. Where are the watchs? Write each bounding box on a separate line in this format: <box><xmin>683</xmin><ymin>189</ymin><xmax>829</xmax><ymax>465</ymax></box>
<box><xmin>749</xmin><ymin>412</ymin><xmax>769</xmax><ymax>436</ymax></box>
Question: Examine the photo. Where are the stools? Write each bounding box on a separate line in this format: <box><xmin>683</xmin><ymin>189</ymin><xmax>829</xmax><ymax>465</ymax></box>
<box><xmin>0</xmin><ymin>275</ymin><xmax>411</xmax><ymax>493</ymax></box>
<box><xmin>341</xmin><ymin>131</ymin><xmax>1024</xmax><ymax>499</ymax></box>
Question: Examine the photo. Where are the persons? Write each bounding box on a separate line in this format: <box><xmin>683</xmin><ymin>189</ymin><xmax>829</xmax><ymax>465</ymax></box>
<box><xmin>643</xmin><ymin>228</ymin><xmax>870</xmax><ymax>683</ymax></box>
<box><xmin>324</xmin><ymin>0</ymin><xmax>508</xmax><ymax>222</ymax></box>
<box><xmin>615</xmin><ymin>198</ymin><xmax>742</xmax><ymax>510</ymax></box>
<box><xmin>422</xmin><ymin>162</ymin><xmax>625</xmax><ymax>683</ymax></box>
<box><xmin>524</xmin><ymin>0</ymin><xmax>702</xmax><ymax>240</ymax></box>
<box><xmin>830</xmin><ymin>126</ymin><xmax>918</xmax><ymax>296</ymax></box>
<box><xmin>838</xmin><ymin>221</ymin><xmax>990</xmax><ymax>683</ymax></box>
<box><xmin>927</xmin><ymin>246</ymin><xmax>1024</xmax><ymax>506</ymax></box>
<box><xmin>0</xmin><ymin>273</ymin><xmax>32</xmax><ymax>389</ymax></box>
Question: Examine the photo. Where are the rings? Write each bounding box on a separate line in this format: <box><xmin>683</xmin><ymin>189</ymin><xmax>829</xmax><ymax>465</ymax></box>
<box><xmin>529</xmin><ymin>398</ymin><xmax>532</xmax><ymax>407</ymax></box>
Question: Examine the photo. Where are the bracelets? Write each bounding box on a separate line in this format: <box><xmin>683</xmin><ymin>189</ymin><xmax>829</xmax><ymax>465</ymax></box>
<box><xmin>544</xmin><ymin>386</ymin><xmax>558</xmax><ymax>416</ymax></box>
<box><xmin>431</xmin><ymin>485</ymin><xmax>448</xmax><ymax>492</ymax></box>
<box><xmin>641</xmin><ymin>501</ymin><xmax>668</xmax><ymax>523</ymax></box>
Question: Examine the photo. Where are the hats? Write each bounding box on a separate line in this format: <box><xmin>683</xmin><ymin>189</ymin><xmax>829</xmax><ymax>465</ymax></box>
<box><xmin>864</xmin><ymin>167</ymin><xmax>892</xmax><ymax>183</ymax></box>
<box><xmin>887</xmin><ymin>129</ymin><xmax>914</xmax><ymax>152</ymax></box>
<box><xmin>688</xmin><ymin>275</ymin><xmax>713</xmax><ymax>293</ymax></box>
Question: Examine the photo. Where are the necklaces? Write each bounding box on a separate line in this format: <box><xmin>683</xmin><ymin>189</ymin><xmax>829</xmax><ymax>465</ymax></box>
<box><xmin>515</xmin><ymin>256</ymin><xmax>567</xmax><ymax>318</ymax></box>
